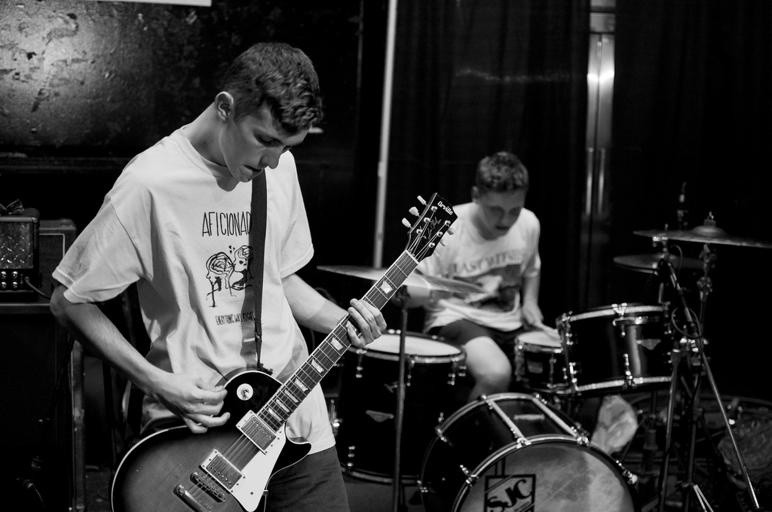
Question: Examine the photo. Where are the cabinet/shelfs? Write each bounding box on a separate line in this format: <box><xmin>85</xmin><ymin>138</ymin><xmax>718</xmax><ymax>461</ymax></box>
<box><xmin>2</xmin><ymin>232</ymin><xmax>121</xmax><ymax>512</ymax></box>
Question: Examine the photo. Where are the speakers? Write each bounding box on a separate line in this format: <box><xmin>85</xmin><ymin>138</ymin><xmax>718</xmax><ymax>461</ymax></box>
<box><xmin>0</xmin><ymin>302</ymin><xmax>87</xmax><ymax>512</ymax></box>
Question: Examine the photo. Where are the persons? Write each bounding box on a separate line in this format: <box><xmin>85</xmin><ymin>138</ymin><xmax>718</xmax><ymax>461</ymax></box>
<box><xmin>49</xmin><ymin>42</ymin><xmax>387</xmax><ymax>512</ymax></box>
<box><xmin>406</xmin><ymin>151</ymin><xmax>547</xmax><ymax>403</ymax></box>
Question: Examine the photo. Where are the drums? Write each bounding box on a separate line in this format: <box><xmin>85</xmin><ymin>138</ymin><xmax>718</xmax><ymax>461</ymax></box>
<box><xmin>514</xmin><ymin>328</ymin><xmax>569</xmax><ymax>396</ymax></box>
<box><xmin>418</xmin><ymin>391</ymin><xmax>642</xmax><ymax>511</ymax></box>
<box><xmin>556</xmin><ymin>301</ymin><xmax>671</xmax><ymax>397</ymax></box>
<box><xmin>331</xmin><ymin>331</ymin><xmax>468</xmax><ymax>487</ymax></box>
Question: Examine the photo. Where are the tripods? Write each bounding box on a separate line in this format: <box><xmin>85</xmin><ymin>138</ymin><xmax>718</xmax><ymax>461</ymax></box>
<box><xmin>640</xmin><ymin>278</ymin><xmax>714</xmax><ymax>512</ymax></box>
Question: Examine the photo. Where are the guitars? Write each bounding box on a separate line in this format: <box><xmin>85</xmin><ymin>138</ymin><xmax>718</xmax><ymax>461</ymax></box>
<box><xmin>108</xmin><ymin>193</ymin><xmax>456</xmax><ymax>511</ymax></box>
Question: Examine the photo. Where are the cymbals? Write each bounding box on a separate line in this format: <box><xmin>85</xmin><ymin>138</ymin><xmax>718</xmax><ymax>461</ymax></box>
<box><xmin>633</xmin><ymin>228</ymin><xmax>771</xmax><ymax>250</ymax></box>
<box><xmin>316</xmin><ymin>264</ymin><xmax>484</xmax><ymax>293</ymax></box>
<box><xmin>612</xmin><ymin>251</ymin><xmax>715</xmax><ymax>272</ymax></box>
